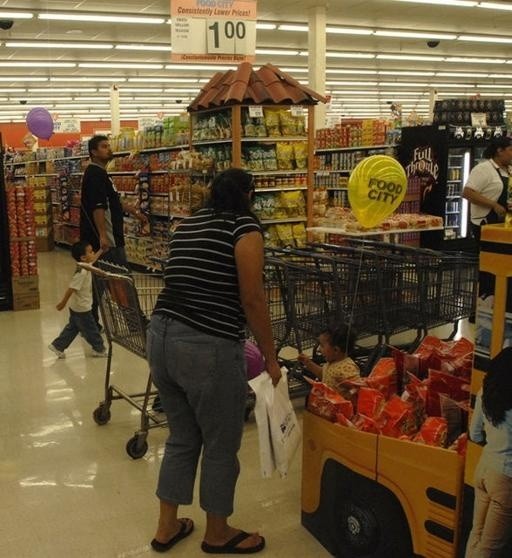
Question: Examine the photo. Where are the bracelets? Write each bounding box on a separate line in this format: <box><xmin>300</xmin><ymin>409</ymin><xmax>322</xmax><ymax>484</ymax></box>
<box><xmin>135</xmin><ymin>209</ymin><xmax>142</xmax><ymax>217</ymax></box>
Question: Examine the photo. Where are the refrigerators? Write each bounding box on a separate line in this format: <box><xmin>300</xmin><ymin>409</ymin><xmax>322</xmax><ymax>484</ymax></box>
<box><xmin>394</xmin><ymin>138</ymin><xmax>499</xmax><ymax>272</ymax></box>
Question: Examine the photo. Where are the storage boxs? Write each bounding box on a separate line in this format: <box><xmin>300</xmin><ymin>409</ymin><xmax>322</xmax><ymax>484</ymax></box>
<box><xmin>13</xmin><ymin>280</ymin><xmax>40</xmax><ymax>310</ymax></box>
<box><xmin>301</xmin><ymin>407</ymin><xmax>465</xmax><ymax>558</ymax></box>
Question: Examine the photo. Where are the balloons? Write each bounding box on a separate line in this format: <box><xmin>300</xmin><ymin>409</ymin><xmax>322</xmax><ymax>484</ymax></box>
<box><xmin>24</xmin><ymin>104</ymin><xmax>54</xmax><ymax>140</ymax></box>
<box><xmin>341</xmin><ymin>151</ymin><xmax>409</xmax><ymax>236</ymax></box>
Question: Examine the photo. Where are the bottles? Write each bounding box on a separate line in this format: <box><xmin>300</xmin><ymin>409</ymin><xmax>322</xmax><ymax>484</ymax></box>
<box><xmin>447</xmin><ymin>170</ymin><xmax>458</xmax><ymax>227</ymax></box>
<box><xmin>119</xmin><ymin>123</ymin><xmax>161</xmax><ymax>150</ymax></box>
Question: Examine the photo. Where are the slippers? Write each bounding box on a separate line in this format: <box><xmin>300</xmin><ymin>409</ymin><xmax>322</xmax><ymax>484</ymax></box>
<box><xmin>201</xmin><ymin>529</ymin><xmax>265</xmax><ymax>554</ymax></box>
<box><xmin>150</xmin><ymin>518</ymin><xmax>194</xmax><ymax>552</ymax></box>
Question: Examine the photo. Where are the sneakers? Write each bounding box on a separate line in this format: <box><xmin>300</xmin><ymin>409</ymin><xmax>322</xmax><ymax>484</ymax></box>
<box><xmin>97</xmin><ymin>324</ymin><xmax>105</xmax><ymax>333</ymax></box>
<box><xmin>92</xmin><ymin>351</ymin><xmax>109</xmax><ymax>357</ymax></box>
<box><xmin>48</xmin><ymin>344</ymin><xmax>65</xmax><ymax>358</ymax></box>
<box><xmin>129</xmin><ymin>319</ymin><xmax>151</xmax><ymax>332</ymax></box>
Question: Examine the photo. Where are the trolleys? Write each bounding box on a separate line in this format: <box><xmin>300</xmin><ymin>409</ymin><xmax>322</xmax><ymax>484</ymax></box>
<box><xmin>149</xmin><ymin>238</ymin><xmax>482</xmax><ymax>424</ymax></box>
<box><xmin>74</xmin><ymin>260</ymin><xmax>169</xmax><ymax>459</ymax></box>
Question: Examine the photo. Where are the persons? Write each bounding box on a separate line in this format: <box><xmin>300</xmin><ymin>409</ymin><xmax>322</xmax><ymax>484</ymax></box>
<box><xmin>49</xmin><ymin>240</ymin><xmax>109</xmax><ymax>358</ymax></box>
<box><xmin>463</xmin><ymin>344</ymin><xmax>511</xmax><ymax>558</ymax></box>
<box><xmin>294</xmin><ymin>321</ymin><xmax>362</xmax><ymax>416</ymax></box>
<box><xmin>145</xmin><ymin>167</ymin><xmax>282</xmax><ymax>554</ymax></box>
<box><xmin>462</xmin><ymin>136</ymin><xmax>511</xmax><ymax>301</ymax></box>
<box><xmin>78</xmin><ymin>134</ymin><xmax>153</xmax><ymax>334</ymax></box>
